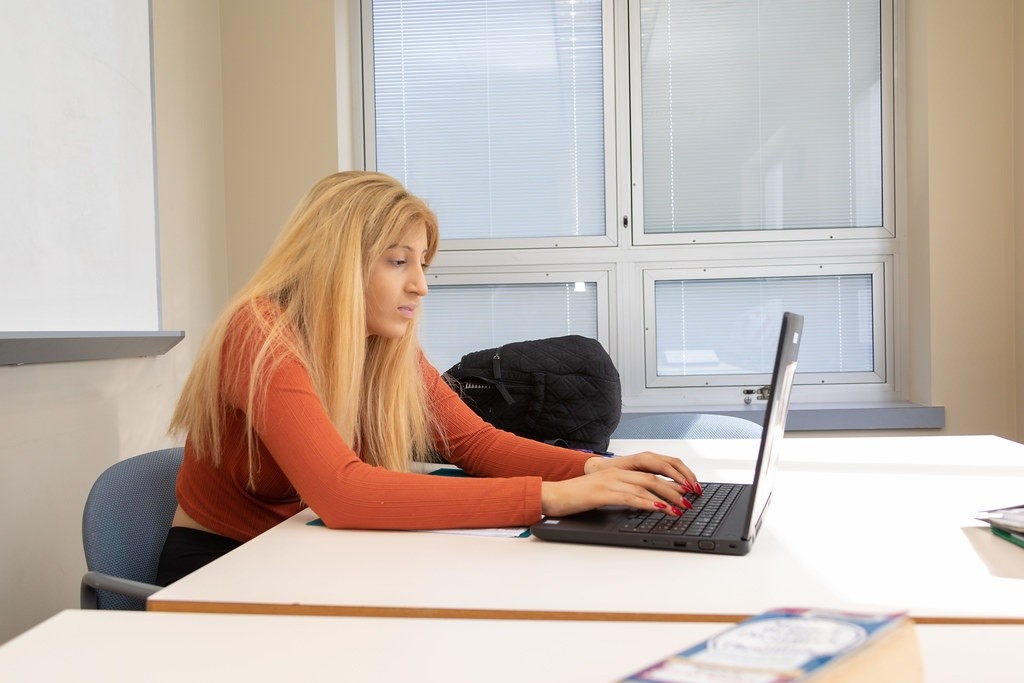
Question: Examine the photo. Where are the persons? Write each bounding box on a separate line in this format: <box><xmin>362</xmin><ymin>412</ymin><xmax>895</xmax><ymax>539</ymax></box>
<box><xmin>152</xmin><ymin>169</ymin><xmax>703</xmax><ymax>587</ymax></box>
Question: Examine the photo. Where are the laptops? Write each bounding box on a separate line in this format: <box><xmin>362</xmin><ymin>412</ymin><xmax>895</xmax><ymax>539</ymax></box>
<box><xmin>531</xmin><ymin>312</ymin><xmax>805</xmax><ymax>556</ymax></box>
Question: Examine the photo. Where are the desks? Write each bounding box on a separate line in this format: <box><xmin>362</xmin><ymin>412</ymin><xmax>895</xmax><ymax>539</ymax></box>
<box><xmin>0</xmin><ymin>446</ymin><xmax>1021</xmax><ymax>676</ymax></box>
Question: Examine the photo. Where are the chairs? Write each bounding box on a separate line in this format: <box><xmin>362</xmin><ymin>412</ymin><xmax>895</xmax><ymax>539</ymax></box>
<box><xmin>77</xmin><ymin>448</ymin><xmax>191</xmax><ymax>614</ymax></box>
